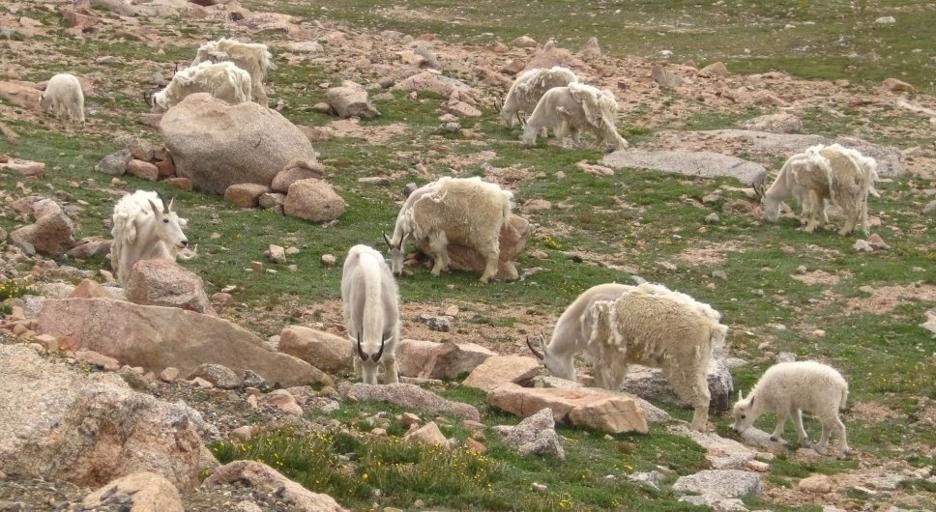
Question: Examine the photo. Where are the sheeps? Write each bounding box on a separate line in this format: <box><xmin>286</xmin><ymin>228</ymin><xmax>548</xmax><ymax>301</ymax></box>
<box><xmin>752</xmin><ymin>143</ymin><xmax>881</xmax><ymax>237</ymax></box>
<box><xmin>732</xmin><ymin>360</ymin><xmax>850</xmax><ymax>459</ymax></box>
<box><xmin>494</xmin><ymin>65</ymin><xmax>578</xmax><ymax>129</ymax></box>
<box><xmin>37</xmin><ymin>74</ymin><xmax>86</xmax><ymax>130</ymax></box>
<box><xmin>516</xmin><ymin>82</ymin><xmax>629</xmax><ymax>152</ymax></box>
<box><xmin>383</xmin><ymin>176</ymin><xmax>514</xmax><ymax>287</ymax></box>
<box><xmin>527</xmin><ymin>282</ymin><xmax>729</xmax><ymax>433</ymax></box>
<box><xmin>111</xmin><ymin>190</ymin><xmax>198</xmax><ymax>284</ymax></box>
<box><xmin>340</xmin><ymin>244</ymin><xmax>400</xmax><ymax>386</ymax></box>
<box><xmin>141</xmin><ymin>37</ymin><xmax>277</xmax><ymax>129</ymax></box>
